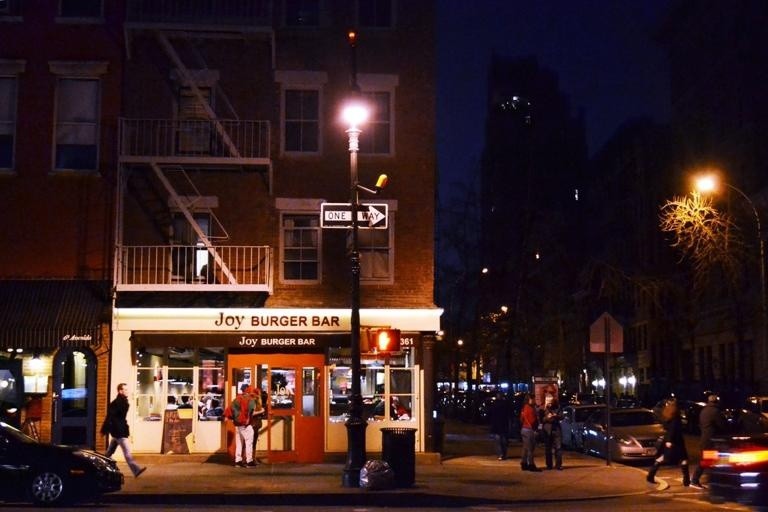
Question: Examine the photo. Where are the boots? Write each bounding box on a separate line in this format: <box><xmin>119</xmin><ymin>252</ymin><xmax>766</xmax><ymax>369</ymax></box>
<box><xmin>647</xmin><ymin>461</ymin><xmax>660</xmax><ymax>484</ymax></box>
<box><xmin>680</xmin><ymin>464</ymin><xmax>690</xmax><ymax>486</ymax></box>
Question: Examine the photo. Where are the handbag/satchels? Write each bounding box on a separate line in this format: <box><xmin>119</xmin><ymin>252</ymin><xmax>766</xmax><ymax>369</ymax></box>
<box><xmin>534</xmin><ymin>429</ymin><xmax>545</xmax><ymax>443</ymax></box>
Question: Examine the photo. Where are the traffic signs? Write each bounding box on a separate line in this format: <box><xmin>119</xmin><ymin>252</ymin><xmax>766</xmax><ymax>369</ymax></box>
<box><xmin>320</xmin><ymin>202</ymin><xmax>388</xmax><ymax>230</ymax></box>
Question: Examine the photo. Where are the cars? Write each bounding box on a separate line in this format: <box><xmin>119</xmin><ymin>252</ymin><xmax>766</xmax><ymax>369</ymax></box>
<box><xmin>648</xmin><ymin>393</ymin><xmax>768</xmax><ymax>432</ymax></box>
<box><xmin>700</xmin><ymin>431</ymin><xmax>768</xmax><ymax>507</ymax></box>
<box><xmin>582</xmin><ymin>406</ymin><xmax>671</xmax><ymax>463</ymax></box>
<box><xmin>439</xmin><ymin>382</ymin><xmax>641</xmax><ymax>449</ymax></box>
<box><xmin>0</xmin><ymin>421</ymin><xmax>125</xmax><ymax>508</ymax></box>
<box><xmin>60</xmin><ymin>388</ymin><xmax>90</xmax><ymax>442</ymax></box>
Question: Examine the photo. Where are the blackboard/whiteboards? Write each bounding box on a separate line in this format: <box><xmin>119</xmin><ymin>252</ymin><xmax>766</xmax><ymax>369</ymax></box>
<box><xmin>162</xmin><ymin>409</ymin><xmax>194</xmax><ymax>455</ymax></box>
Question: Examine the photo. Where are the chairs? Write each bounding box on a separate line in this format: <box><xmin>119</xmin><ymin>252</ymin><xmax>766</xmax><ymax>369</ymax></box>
<box><xmin>148</xmin><ymin>395</ymin><xmax>227</xmax><ymax>429</ymax></box>
<box><xmin>329</xmin><ymin>395</ymin><xmax>395</xmax><ymax>421</ymax></box>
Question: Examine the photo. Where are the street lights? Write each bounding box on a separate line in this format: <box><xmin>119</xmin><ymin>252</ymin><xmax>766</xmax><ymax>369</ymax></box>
<box><xmin>693</xmin><ymin>173</ymin><xmax>766</xmax><ymax>406</ymax></box>
<box><xmin>333</xmin><ymin>24</ymin><xmax>379</xmax><ymax>487</ymax></box>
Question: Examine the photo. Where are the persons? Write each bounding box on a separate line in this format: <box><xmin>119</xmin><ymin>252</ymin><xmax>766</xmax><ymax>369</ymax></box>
<box><xmin>517</xmin><ymin>393</ymin><xmax>540</xmax><ymax>473</ymax></box>
<box><xmin>336</xmin><ymin>382</ymin><xmax>408</xmax><ymax>420</ymax></box>
<box><xmin>222</xmin><ymin>385</ymin><xmax>268</xmax><ymax>469</ymax></box>
<box><xmin>163</xmin><ymin>384</ymin><xmax>222</xmax><ymax>422</ymax></box>
<box><xmin>686</xmin><ymin>389</ymin><xmax>731</xmax><ymax>488</ymax></box>
<box><xmin>491</xmin><ymin>390</ymin><xmax>511</xmax><ymax>462</ymax></box>
<box><xmin>647</xmin><ymin>398</ymin><xmax>691</xmax><ymax>487</ymax></box>
<box><xmin>100</xmin><ymin>382</ymin><xmax>149</xmax><ymax>480</ymax></box>
<box><xmin>537</xmin><ymin>393</ymin><xmax>564</xmax><ymax>473</ymax></box>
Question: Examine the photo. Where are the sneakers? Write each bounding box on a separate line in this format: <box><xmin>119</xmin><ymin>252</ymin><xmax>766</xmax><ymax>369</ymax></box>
<box><xmin>234</xmin><ymin>460</ymin><xmax>247</xmax><ymax>467</ymax></box>
<box><xmin>136</xmin><ymin>467</ymin><xmax>147</xmax><ymax>477</ymax></box>
<box><xmin>528</xmin><ymin>465</ymin><xmax>542</xmax><ymax>471</ymax></box>
<box><xmin>689</xmin><ymin>481</ymin><xmax>705</xmax><ymax>489</ymax></box>
<box><xmin>521</xmin><ymin>463</ymin><xmax>529</xmax><ymax>470</ymax></box>
<box><xmin>247</xmin><ymin>458</ymin><xmax>261</xmax><ymax>466</ymax></box>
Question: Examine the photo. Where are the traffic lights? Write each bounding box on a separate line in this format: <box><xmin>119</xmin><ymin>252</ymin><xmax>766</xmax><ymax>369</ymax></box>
<box><xmin>375</xmin><ymin>328</ymin><xmax>401</xmax><ymax>353</ymax></box>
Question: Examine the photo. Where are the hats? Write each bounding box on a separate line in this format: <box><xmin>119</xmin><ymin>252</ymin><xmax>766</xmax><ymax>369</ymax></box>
<box><xmin>544</xmin><ymin>394</ymin><xmax>555</xmax><ymax>411</ymax></box>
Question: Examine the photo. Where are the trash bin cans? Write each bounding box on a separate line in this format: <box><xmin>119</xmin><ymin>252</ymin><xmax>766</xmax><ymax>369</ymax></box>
<box><xmin>379</xmin><ymin>427</ymin><xmax>418</xmax><ymax>487</ymax></box>
